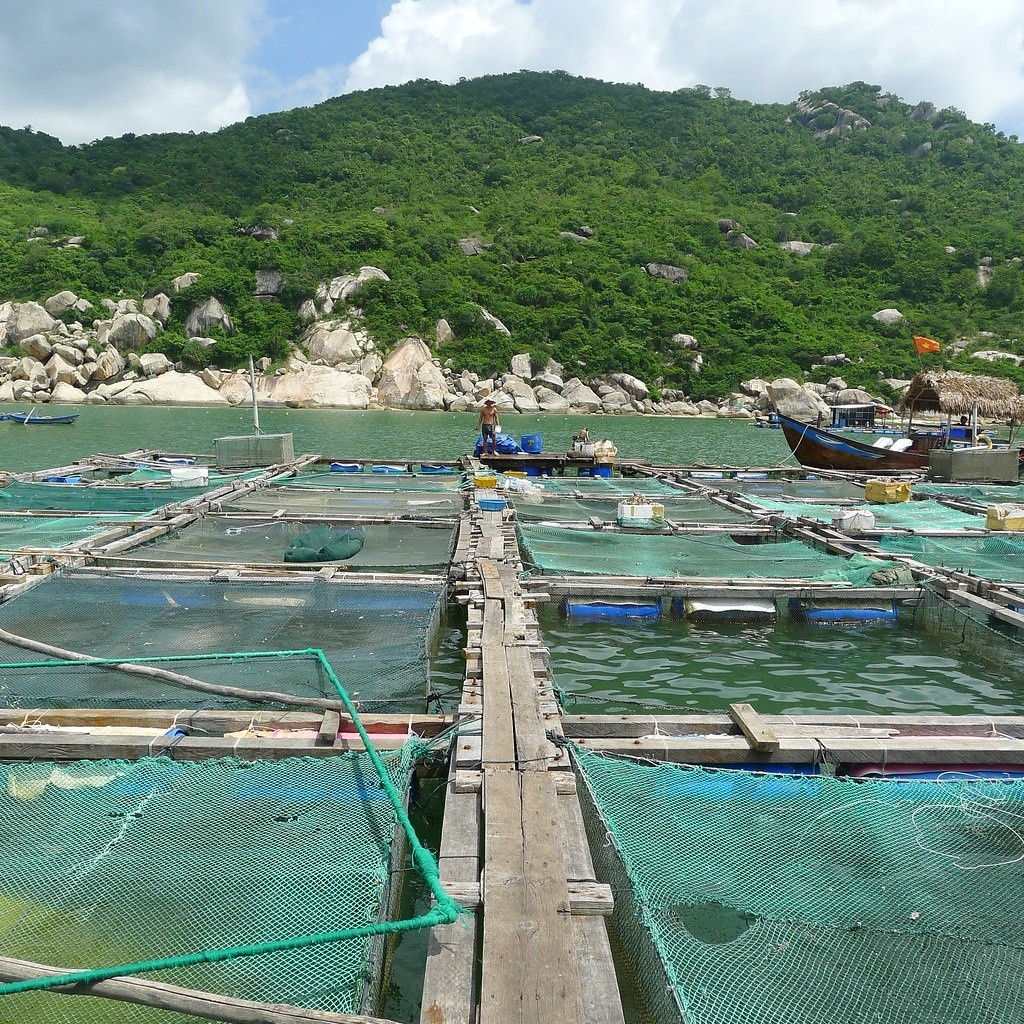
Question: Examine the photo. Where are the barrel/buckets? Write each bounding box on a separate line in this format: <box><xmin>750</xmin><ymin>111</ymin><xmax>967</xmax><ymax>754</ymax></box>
<box><xmin>521</xmin><ymin>434</ymin><xmax>543</xmax><ymax>454</ymax></box>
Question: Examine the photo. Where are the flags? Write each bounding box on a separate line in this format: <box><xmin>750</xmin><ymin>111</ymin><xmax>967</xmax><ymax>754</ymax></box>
<box><xmin>913</xmin><ymin>336</ymin><xmax>940</xmax><ymax>355</ymax></box>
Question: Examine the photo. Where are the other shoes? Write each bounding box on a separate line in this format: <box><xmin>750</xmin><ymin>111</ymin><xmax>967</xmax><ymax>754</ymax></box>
<box><xmin>491</xmin><ymin>450</ymin><xmax>499</xmax><ymax>456</ymax></box>
<box><xmin>480</xmin><ymin>450</ymin><xmax>488</xmax><ymax>456</ymax></box>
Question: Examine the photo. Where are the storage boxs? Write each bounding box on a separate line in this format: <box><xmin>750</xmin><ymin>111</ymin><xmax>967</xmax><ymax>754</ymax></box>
<box><xmin>864</xmin><ymin>478</ymin><xmax>911</xmax><ymax>506</ymax></box>
<box><xmin>942</xmin><ymin>428</ymin><xmax>966</xmax><ymax>439</ymax></box>
<box><xmin>502</xmin><ymin>433</ymin><xmax>622</xmax><ymax>480</ymax></box>
<box><xmin>169</xmin><ymin>467</ymin><xmax>209</xmax><ymax>489</ymax></box>
<box><xmin>616</xmin><ymin>501</ymin><xmax>666</xmax><ymax>530</ymax></box>
<box><xmin>474</xmin><ymin>476</ymin><xmax>497</xmax><ymax>489</ymax></box>
<box><xmin>986</xmin><ymin>503</ymin><xmax>1024</xmax><ymax>532</ymax></box>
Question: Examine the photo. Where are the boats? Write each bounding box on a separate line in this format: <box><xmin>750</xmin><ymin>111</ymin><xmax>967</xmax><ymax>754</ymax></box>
<box><xmin>477</xmin><ymin>452</ymin><xmax>652</xmax><ymax>472</ymax></box>
<box><xmin>756</xmin><ymin>411</ymin><xmax>792</xmax><ymax>424</ymax></box>
<box><xmin>779</xmin><ymin>373</ymin><xmax>1024</xmax><ymax>469</ymax></box>
<box><xmin>8</xmin><ymin>412</ymin><xmax>80</xmax><ymax>423</ymax></box>
<box><xmin>0</xmin><ymin>411</ymin><xmax>26</xmax><ymax>420</ymax></box>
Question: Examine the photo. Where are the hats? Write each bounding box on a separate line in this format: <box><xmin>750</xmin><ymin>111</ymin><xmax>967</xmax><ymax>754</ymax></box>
<box><xmin>484</xmin><ymin>396</ymin><xmax>496</xmax><ymax>403</ymax></box>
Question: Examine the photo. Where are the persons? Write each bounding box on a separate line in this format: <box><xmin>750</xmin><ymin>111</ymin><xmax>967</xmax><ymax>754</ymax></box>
<box><xmin>574</xmin><ymin>427</ymin><xmax>591</xmax><ymax>442</ymax></box>
<box><xmin>959</xmin><ymin>415</ymin><xmax>969</xmax><ymax>426</ymax></box>
<box><xmin>475</xmin><ymin>400</ymin><xmax>500</xmax><ymax>456</ymax></box>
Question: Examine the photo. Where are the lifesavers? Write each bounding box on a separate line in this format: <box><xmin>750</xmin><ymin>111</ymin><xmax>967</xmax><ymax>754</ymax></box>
<box><xmin>975</xmin><ymin>434</ymin><xmax>992</xmax><ymax>449</ymax></box>
